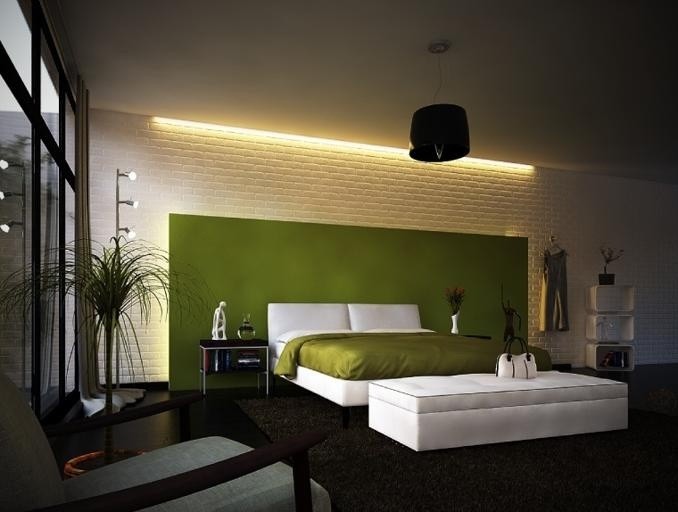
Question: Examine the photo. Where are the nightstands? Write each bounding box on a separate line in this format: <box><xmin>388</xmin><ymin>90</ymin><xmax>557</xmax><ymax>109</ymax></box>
<box><xmin>201</xmin><ymin>338</ymin><xmax>269</xmax><ymax>398</ymax></box>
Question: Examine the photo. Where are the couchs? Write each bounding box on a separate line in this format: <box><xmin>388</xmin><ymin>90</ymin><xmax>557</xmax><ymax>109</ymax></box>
<box><xmin>368</xmin><ymin>373</ymin><xmax>627</xmax><ymax>453</ymax></box>
<box><xmin>0</xmin><ymin>370</ymin><xmax>332</xmax><ymax>512</ymax></box>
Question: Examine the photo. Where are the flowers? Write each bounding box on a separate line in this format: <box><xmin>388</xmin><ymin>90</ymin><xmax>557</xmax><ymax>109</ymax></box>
<box><xmin>445</xmin><ymin>288</ymin><xmax>464</xmax><ymax>314</ymax></box>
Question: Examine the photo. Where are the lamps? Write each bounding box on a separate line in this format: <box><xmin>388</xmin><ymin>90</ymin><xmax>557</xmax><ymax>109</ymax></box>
<box><xmin>0</xmin><ymin>160</ymin><xmax>26</xmax><ymax>386</ymax></box>
<box><xmin>116</xmin><ymin>170</ymin><xmax>138</xmax><ymax>387</ymax></box>
<box><xmin>409</xmin><ymin>40</ymin><xmax>470</xmax><ymax>162</ymax></box>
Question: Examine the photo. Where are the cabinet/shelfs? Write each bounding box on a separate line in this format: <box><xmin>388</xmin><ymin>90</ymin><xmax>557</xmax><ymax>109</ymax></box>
<box><xmin>587</xmin><ymin>284</ymin><xmax>636</xmax><ymax>371</ymax></box>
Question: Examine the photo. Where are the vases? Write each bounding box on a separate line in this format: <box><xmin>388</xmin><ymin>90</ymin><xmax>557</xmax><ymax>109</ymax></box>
<box><xmin>451</xmin><ymin>311</ymin><xmax>461</xmax><ymax>334</ymax></box>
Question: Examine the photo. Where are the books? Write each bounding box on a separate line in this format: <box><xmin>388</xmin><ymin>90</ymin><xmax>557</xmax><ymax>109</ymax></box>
<box><xmin>203</xmin><ymin>349</ymin><xmax>232</xmax><ymax>373</ymax></box>
<box><xmin>237</xmin><ymin>350</ymin><xmax>261</xmax><ymax>370</ymax></box>
<box><xmin>601</xmin><ymin>352</ymin><xmax>629</xmax><ymax>367</ymax></box>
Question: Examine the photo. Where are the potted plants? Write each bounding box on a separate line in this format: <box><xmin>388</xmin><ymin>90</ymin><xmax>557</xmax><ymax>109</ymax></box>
<box><xmin>598</xmin><ymin>244</ymin><xmax>623</xmax><ymax>284</ymax></box>
<box><xmin>0</xmin><ymin>234</ymin><xmax>217</xmax><ymax>478</ymax></box>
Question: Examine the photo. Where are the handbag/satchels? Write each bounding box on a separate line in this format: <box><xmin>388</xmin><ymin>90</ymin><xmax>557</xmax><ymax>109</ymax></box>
<box><xmin>495</xmin><ymin>335</ymin><xmax>536</xmax><ymax>380</ymax></box>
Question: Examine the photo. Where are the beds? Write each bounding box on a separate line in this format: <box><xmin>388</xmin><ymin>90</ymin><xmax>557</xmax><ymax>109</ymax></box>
<box><xmin>268</xmin><ymin>303</ymin><xmax>551</xmax><ymax>406</ymax></box>
